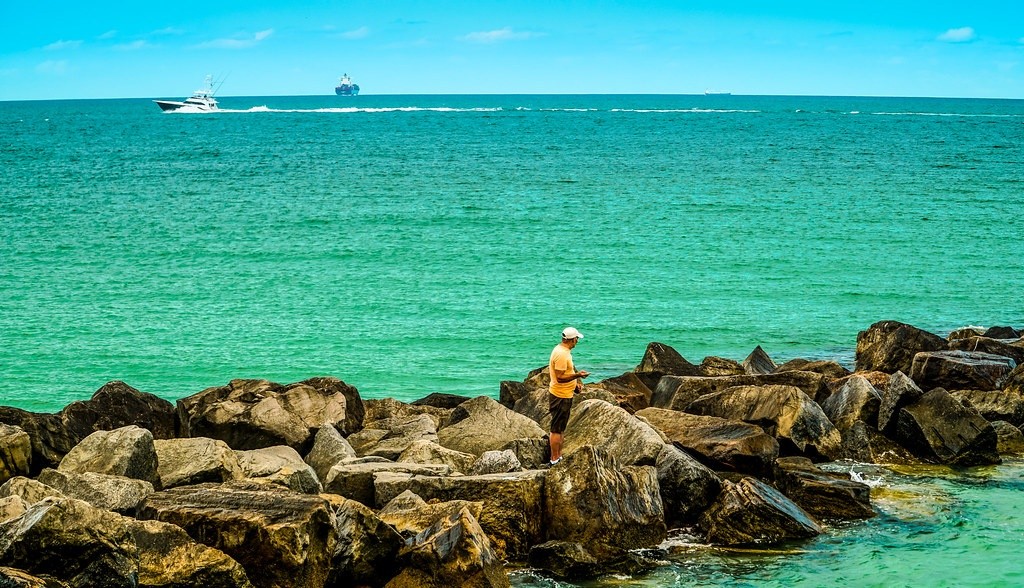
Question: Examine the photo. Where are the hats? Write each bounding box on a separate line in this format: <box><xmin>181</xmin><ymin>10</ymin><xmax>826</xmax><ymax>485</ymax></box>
<box><xmin>562</xmin><ymin>327</ymin><xmax>584</xmax><ymax>339</ymax></box>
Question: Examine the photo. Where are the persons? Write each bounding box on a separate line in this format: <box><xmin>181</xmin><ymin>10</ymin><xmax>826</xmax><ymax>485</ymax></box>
<box><xmin>548</xmin><ymin>327</ymin><xmax>592</xmax><ymax>468</ymax></box>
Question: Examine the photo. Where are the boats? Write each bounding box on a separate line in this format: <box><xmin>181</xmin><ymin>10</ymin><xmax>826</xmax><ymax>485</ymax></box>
<box><xmin>152</xmin><ymin>69</ymin><xmax>231</xmax><ymax>112</ymax></box>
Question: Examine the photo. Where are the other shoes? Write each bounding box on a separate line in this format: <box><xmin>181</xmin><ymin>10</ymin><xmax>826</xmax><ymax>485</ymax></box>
<box><xmin>548</xmin><ymin>456</ymin><xmax>563</xmax><ymax>468</ymax></box>
